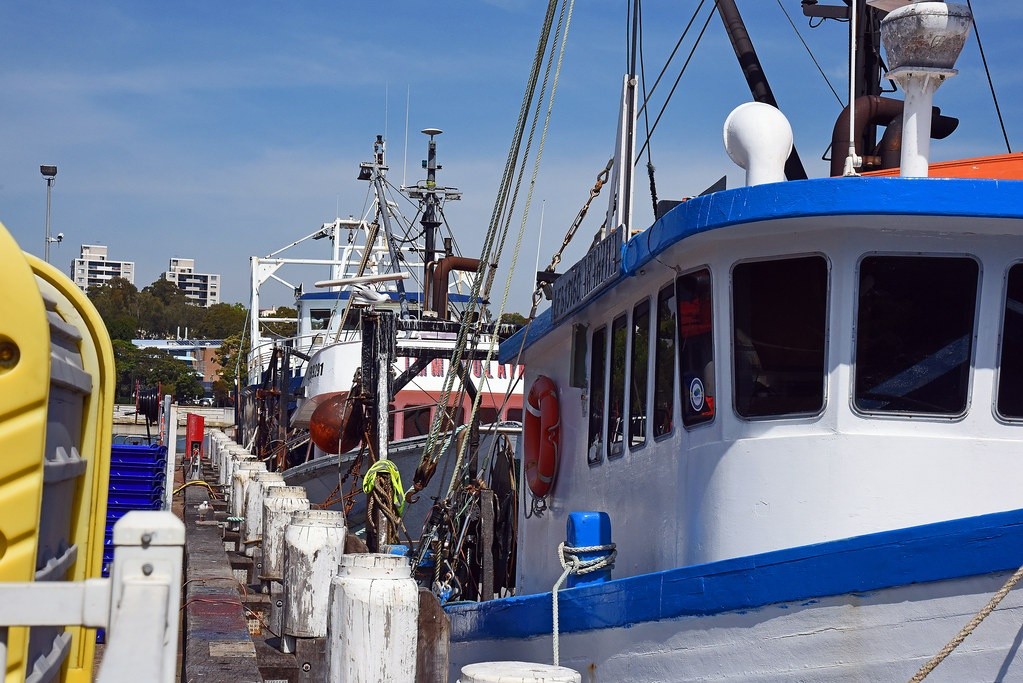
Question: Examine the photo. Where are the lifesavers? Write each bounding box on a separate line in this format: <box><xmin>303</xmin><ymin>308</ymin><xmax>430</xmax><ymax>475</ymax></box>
<box><xmin>521</xmin><ymin>374</ymin><xmax>559</xmax><ymax>500</ymax></box>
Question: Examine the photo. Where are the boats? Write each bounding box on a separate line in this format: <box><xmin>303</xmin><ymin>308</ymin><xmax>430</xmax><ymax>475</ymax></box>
<box><xmin>232</xmin><ymin>1</ymin><xmax>1023</xmax><ymax>682</ymax></box>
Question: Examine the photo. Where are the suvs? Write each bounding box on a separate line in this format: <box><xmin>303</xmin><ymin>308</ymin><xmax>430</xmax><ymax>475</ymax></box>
<box><xmin>195</xmin><ymin>397</ymin><xmax>215</xmax><ymax>406</ymax></box>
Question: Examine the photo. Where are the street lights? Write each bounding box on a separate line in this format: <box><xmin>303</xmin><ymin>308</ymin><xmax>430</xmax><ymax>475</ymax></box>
<box><xmin>38</xmin><ymin>163</ymin><xmax>64</xmax><ymax>263</ymax></box>
